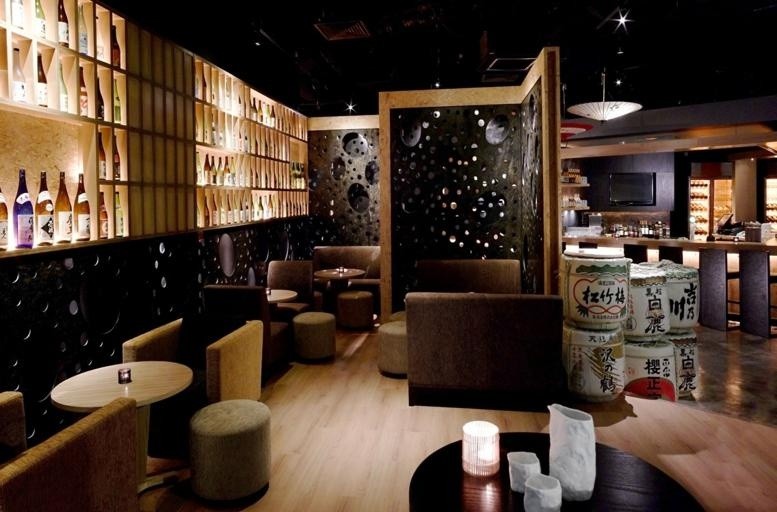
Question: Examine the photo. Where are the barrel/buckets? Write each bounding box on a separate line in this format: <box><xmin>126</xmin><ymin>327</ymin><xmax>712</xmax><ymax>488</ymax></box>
<box><xmin>639</xmin><ymin>259</ymin><xmax>701</xmax><ymax>334</ymax></box>
<box><xmin>562</xmin><ymin>321</ymin><xmax>626</xmax><ymax>402</ymax></box>
<box><xmin>624</xmin><ymin>340</ymin><xmax>678</xmax><ymax>402</ymax></box>
<box><xmin>561</xmin><ymin>247</ymin><xmax>632</xmax><ymax>330</ymax></box>
<box><xmin>669</xmin><ymin>330</ymin><xmax>700</xmax><ymax>398</ymax></box>
<box><xmin>625</xmin><ymin>263</ymin><xmax>671</xmax><ymax>342</ymax></box>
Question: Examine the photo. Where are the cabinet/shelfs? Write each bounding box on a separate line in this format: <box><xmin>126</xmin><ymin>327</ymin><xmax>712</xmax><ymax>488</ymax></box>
<box><xmin>0</xmin><ymin>1</ymin><xmax>312</xmax><ymax>261</ymax></box>
<box><xmin>674</xmin><ymin>150</ymin><xmax>776</xmax><ymax>240</ymax></box>
<box><xmin>561</xmin><ymin>183</ymin><xmax>590</xmax><ymax>211</ymax></box>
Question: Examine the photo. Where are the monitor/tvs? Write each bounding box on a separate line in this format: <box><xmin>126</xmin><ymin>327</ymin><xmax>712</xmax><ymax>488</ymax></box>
<box><xmin>715</xmin><ymin>213</ymin><xmax>733</xmax><ymax>227</ymax></box>
<box><xmin>608</xmin><ymin>171</ymin><xmax>655</xmax><ymax>207</ymax></box>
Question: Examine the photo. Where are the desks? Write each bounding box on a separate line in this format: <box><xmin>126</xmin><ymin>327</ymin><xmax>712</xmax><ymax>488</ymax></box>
<box><xmin>409</xmin><ymin>432</ymin><xmax>704</xmax><ymax>512</ymax></box>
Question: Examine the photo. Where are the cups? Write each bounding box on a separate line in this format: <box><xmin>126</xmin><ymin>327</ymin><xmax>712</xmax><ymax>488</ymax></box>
<box><xmin>507</xmin><ymin>451</ymin><xmax>540</xmax><ymax>492</ymax></box>
<box><xmin>524</xmin><ymin>474</ymin><xmax>562</xmax><ymax>511</ymax></box>
<box><xmin>548</xmin><ymin>402</ymin><xmax>597</xmax><ymax>501</ymax></box>
<box><xmin>463</xmin><ymin>420</ymin><xmax>500</xmax><ymax>475</ymax></box>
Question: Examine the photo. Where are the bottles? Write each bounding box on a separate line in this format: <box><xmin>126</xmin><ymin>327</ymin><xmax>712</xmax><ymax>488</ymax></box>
<box><xmin>195</xmin><ymin>59</ymin><xmax>307</xmax><ymax>228</ymax></box>
<box><xmin>610</xmin><ymin>220</ymin><xmax>670</xmax><ymax>238</ymax></box>
<box><xmin>0</xmin><ymin>0</ymin><xmax>124</xmax><ymax>251</ymax></box>
<box><xmin>566</xmin><ymin>168</ymin><xmax>580</xmax><ymax>184</ymax></box>
